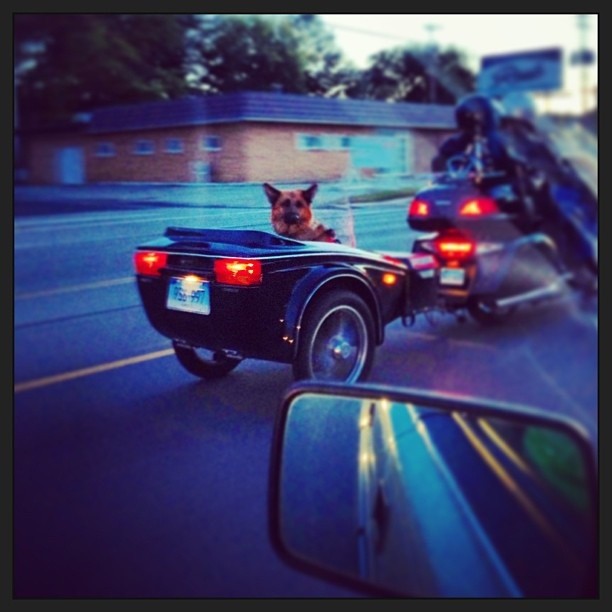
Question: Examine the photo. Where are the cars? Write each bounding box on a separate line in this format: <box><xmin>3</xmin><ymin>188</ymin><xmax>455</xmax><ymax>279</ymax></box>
<box><xmin>357</xmin><ymin>401</ymin><xmax>589</xmax><ymax>602</ymax></box>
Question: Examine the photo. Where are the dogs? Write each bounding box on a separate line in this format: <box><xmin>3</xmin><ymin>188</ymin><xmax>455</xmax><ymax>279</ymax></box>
<box><xmin>263</xmin><ymin>182</ymin><xmax>342</xmax><ymax>243</ymax></box>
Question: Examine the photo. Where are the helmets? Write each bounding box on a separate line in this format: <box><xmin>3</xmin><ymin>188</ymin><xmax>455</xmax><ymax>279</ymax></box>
<box><xmin>501</xmin><ymin>91</ymin><xmax>536</xmax><ymax>130</ymax></box>
<box><xmin>456</xmin><ymin>95</ymin><xmax>493</xmax><ymax>132</ymax></box>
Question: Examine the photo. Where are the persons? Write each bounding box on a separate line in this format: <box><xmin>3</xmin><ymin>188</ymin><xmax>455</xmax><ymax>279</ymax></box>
<box><xmin>429</xmin><ymin>94</ymin><xmax>598</xmax><ymax>300</ymax></box>
<box><xmin>494</xmin><ymin>95</ymin><xmax>598</xmax><ymax>240</ymax></box>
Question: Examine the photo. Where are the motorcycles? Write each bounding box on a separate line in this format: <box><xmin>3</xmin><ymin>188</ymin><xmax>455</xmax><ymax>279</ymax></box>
<box><xmin>132</xmin><ymin>155</ymin><xmax>597</xmax><ymax>380</ymax></box>
